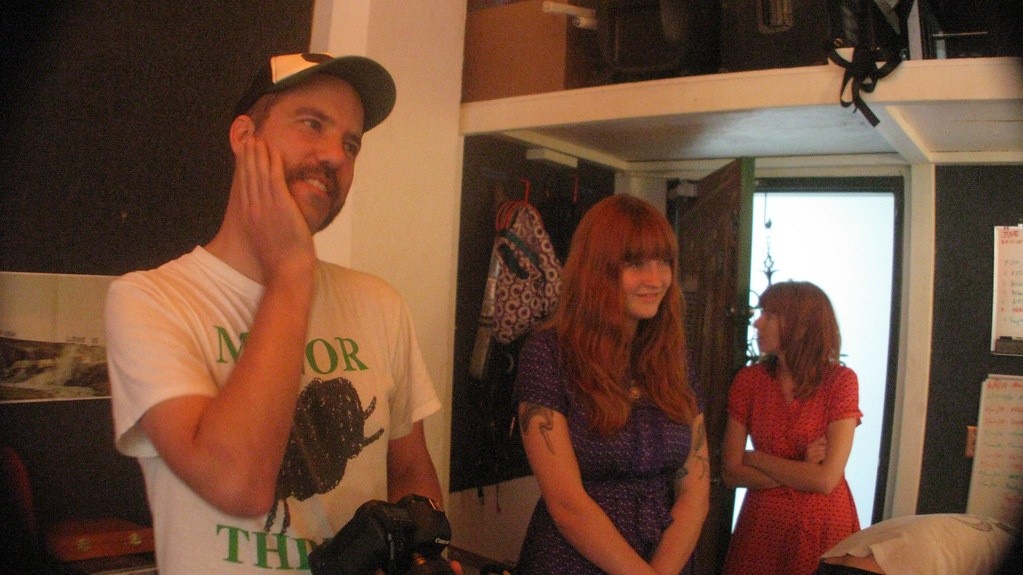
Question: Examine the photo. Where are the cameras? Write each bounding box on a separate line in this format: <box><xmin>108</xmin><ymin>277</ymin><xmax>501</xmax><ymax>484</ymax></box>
<box><xmin>308</xmin><ymin>493</ymin><xmax>455</xmax><ymax>575</ymax></box>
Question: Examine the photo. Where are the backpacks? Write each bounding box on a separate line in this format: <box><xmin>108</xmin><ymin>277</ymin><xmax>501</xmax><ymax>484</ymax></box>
<box><xmin>466</xmin><ymin>331</ymin><xmax>534</xmax><ymax>513</ymax></box>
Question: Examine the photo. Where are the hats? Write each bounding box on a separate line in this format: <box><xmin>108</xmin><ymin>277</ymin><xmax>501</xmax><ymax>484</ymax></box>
<box><xmin>232</xmin><ymin>52</ymin><xmax>396</xmax><ymax>132</ymax></box>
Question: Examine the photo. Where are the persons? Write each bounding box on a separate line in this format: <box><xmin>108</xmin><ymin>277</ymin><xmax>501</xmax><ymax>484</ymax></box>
<box><xmin>717</xmin><ymin>281</ymin><xmax>864</xmax><ymax>575</ymax></box>
<box><xmin>512</xmin><ymin>193</ymin><xmax>711</xmax><ymax>575</ymax></box>
<box><xmin>104</xmin><ymin>52</ymin><xmax>461</xmax><ymax>575</ymax></box>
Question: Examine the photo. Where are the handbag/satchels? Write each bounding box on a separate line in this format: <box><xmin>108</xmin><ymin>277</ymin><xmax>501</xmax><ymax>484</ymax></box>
<box><xmin>493</xmin><ymin>205</ymin><xmax>564</xmax><ymax>345</ymax></box>
